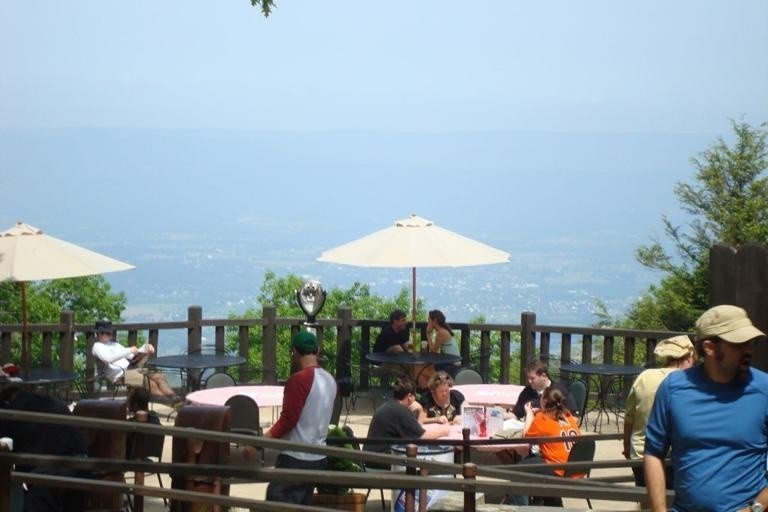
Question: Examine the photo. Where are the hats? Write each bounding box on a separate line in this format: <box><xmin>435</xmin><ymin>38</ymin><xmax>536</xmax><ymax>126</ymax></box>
<box><xmin>290</xmin><ymin>330</ymin><xmax>319</xmax><ymax>351</ymax></box>
<box><xmin>692</xmin><ymin>303</ymin><xmax>767</xmax><ymax>344</ymax></box>
<box><xmin>90</xmin><ymin>319</ymin><xmax>116</xmax><ymax>333</ymax></box>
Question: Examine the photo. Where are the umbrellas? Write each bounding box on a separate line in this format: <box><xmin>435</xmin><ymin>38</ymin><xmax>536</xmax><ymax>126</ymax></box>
<box><xmin>317</xmin><ymin>214</ymin><xmax>511</xmax><ymax>352</ymax></box>
<box><xmin>0</xmin><ymin>222</ymin><xmax>137</xmax><ymax>377</ymax></box>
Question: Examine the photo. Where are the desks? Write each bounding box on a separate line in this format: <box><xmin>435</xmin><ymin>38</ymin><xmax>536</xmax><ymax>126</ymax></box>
<box><xmin>366</xmin><ymin>351</ymin><xmax>647</xmax><ymax>465</ymax></box>
<box><xmin>2</xmin><ymin>354</ymin><xmax>284</xmax><ymax>428</ymax></box>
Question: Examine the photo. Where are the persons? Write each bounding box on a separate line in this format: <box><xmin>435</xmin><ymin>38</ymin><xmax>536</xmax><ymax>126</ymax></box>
<box><xmin>124</xmin><ymin>385</ymin><xmax>161</xmax><ymax>457</ymax></box>
<box><xmin>622</xmin><ymin>335</ymin><xmax>698</xmax><ymax>511</ymax></box>
<box><xmin>91</xmin><ymin>321</ymin><xmax>184</xmax><ymax>409</ymax></box>
<box><xmin>0</xmin><ymin>367</ymin><xmax>23</xmax><ymax>405</ymax></box>
<box><xmin>644</xmin><ymin>303</ymin><xmax>767</xmax><ymax>512</ymax></box>
<box><xmin>2</xmin><ymin>385</ymin><xmax>90</xmax><ymax>511</ymax></box>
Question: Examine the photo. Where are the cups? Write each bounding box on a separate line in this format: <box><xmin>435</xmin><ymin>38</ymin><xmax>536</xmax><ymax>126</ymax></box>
<box><xmin>421</xmin><ymin>341</ymin><xmax>428</xmax><ymax>354</ymax></box>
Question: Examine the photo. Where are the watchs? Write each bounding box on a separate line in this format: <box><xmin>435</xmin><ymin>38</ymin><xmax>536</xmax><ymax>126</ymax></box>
<box><xmin>748</xmin><ymin>501</ymin><xmax>764</xmax><ymax>512</ymax></box>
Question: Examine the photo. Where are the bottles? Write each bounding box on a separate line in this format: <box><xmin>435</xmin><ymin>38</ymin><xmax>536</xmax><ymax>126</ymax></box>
<box><xmin>475</xmin><ymin>409</ymin><xmax>504</xmax><ymax>439</ymax></box>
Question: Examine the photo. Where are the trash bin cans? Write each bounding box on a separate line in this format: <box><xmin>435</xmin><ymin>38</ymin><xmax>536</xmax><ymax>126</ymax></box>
<box><xmin>390</xmin><ymin>444</ymin><xmax>455</xmax><ymax>512</ymax></box>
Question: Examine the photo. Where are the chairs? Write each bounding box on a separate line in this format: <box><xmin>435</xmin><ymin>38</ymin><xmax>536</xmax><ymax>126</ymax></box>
<box><xmin>124</xmin><ymin>412</ymin><xmax>169</xmax><ymax>512</ymax></box>
<box><xmin>343</xmin><ymin>426</ymin><xmax>385</xmax><ymax>512</ymax></box>
<box><xmin>224</xmin><ymin>395</ymin><xmax>265</xmax><ymax>466</ymax></box>
<box><xmin>563</xmin><ymin>440</ymin><xmax>596</xmax><ymax>510</ymax></box>
